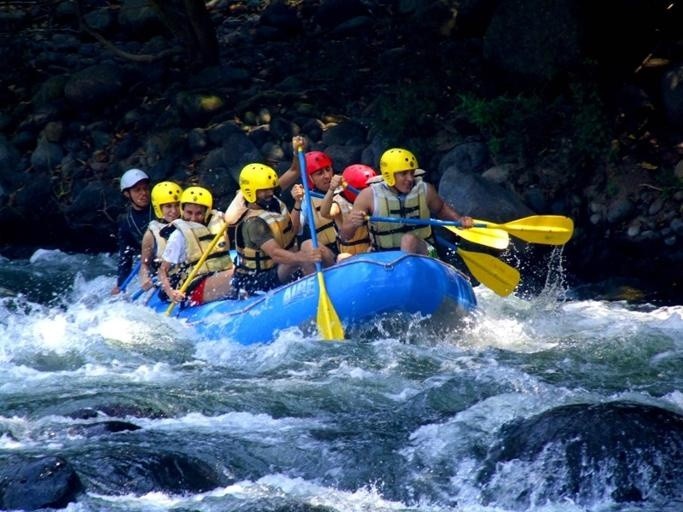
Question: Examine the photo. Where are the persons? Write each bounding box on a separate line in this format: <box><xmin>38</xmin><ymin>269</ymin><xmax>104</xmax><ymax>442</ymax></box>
<box><xmin>232</xmin><ymin>136</ymin><xmax>326</xmax><ymax>300</ymax></box>
<box><xmin>291</xmin><ymin>152</ymin><xmax>342</xmax><ymax>275</ymax></box>
<box><xmin>336</xmin><ymin>148</ymin><xmax>473</xmax><ymax>263</ymax></box>
<box><xmin>321</xmin><ymin>164</ymin><xmax>377</xmax><ymax>264</ymax></box>
<box><xmin>139</xmin><ymin>181</ymin><xmax>183</xmax><ymax>291</ymax></box>
<box><xmin>159</xmin><ymin>185</ymin><xmax>234</xmax><ymax>309</ymax></box>
<box><xmin>110</xmin><ymin>168</ymin><xmax>157</xmax><ymax>297</ymax></box>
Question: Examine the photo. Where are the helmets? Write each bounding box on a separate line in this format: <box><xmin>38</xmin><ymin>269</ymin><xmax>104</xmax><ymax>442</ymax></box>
<box><xmin>120</xmin><ymin>169</ymin><xmax>149</xmax><ymax>191</ymax></box>
<box><xmin>341</xmin><ymin>163</ymin><xmax>376</xmax><ymax>204</ymax></box>
<box><xmin>379</xmin><ymin>148</ymin><xmax>419</xmax><ymax>188</ymax></box>
<box><xmin>178</xmin><ymin>186</ymin><xmax>213</xmax><ymax>224</ymax></box>
<box><xmin>304</xmin><ymin>150</ymin><xmax>333</xmax><ymax>190</ymax></box>
<box><xmin>239</xmin><ymin>163</ymin><xmax>280</xmax><ymax>204</ymax></box>
<box><xmin>150</xmin><ymin>180</ymin><xmax>183</xmax><ymax>221</ymax></box>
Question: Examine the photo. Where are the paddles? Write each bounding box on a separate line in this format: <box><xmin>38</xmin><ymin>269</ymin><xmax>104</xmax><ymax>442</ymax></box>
<box><xmin>297</xmin><ymin>145</ymin><xmax>345</xmax><ymax>342</ymax></box>
<box><xmin>430</xmin><ymin>231</ymin><xmax>520</xmax><ymax>298</ymax></box>
<box><xmin>367</xmin><ymin>214</ymin><xmax>574</xmax><ymax>245</ymax></box>
<box><xmin>342</xmin><ymin>180</ymin><xmax>510</xmax><ymax>250</ymax></box>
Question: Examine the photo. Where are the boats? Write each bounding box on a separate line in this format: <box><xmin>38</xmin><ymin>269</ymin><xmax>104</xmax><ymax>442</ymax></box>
<box><xmin>125</xmin><ymin>252</ymin><xmax>478</xmax><ymax>347</ymax></box>
